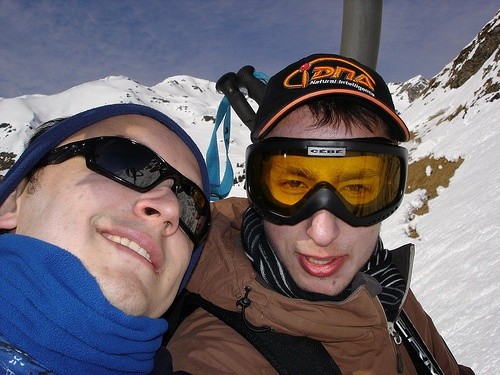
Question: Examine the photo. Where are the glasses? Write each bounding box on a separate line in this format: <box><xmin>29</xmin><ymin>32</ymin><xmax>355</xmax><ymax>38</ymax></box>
<box><xmin>245</xmin><ymin>136</ymin><xmax>407</xmax><ymax>227</ymax></box>
<box><xmin>33</xmin><ymin>135</ymin><xmax>212</xmax><ymax>247</ymax></box>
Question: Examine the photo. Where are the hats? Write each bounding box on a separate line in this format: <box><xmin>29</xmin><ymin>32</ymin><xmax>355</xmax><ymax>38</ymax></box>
<box><xmin>253</xmin><ymin>52</ymin><xmax>410</xmax><ymax>142</ymax></box>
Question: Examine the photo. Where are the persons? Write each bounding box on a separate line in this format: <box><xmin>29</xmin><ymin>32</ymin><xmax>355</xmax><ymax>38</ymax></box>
<box><xmin>165</xmin><ymin>52</ymin><xmax>476</xmax><ymax>375</ymax></box>
<box><xmin>0</xmin><ymin>104</ymin><xmax>212</xmax><ymax>375</ymax></box>
